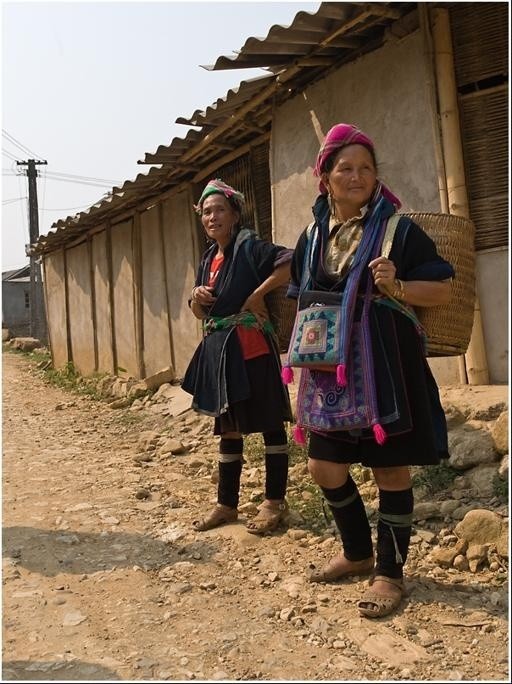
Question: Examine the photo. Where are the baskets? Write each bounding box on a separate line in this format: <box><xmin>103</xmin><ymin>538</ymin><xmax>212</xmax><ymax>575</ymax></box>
<box><xmin>263</xmin><ymin>286</ymin><xmax>298</xmax><ymax>353</ymax></box>
<box><xmin>393</xmin><ymin>211</ymin><xmax>476</xmax><ymax>355</ymax></box>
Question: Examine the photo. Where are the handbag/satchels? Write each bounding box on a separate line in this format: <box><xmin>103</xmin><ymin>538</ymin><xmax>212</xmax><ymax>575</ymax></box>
<box><xmin>288</xmin><ymin>305</ymin><xmax>352</xmax><ymax>368</ymax></box>
<box><xmin>295</xmin><ymin>322</ymin><xmax>377</xmax><ymax>431</ymax></box>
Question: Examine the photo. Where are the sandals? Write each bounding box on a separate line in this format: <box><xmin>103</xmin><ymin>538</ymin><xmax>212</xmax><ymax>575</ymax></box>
<box><xmin>357</xmin><ymin>576</ymin><xmax>407</xmax><ymax>617</ymax></box>
<box><xmin>246</xmin><ymin>500</ymin><xmax>289</xmax><ymax>532</ymax></box>
<box><xmin>192</xmin><ymin>504</ymin><xmax>237</xmax><ymax>532</ymax></box>
<box><xmin>305</xmin><ymin>555</ymin><xmax>374</xmax><ymax>581</ymax></box>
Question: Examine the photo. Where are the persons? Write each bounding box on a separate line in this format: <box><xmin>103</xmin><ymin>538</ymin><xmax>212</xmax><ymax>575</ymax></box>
<box><xmin>280</xmin><ymin>123</ymin><xmax>456</xmax><ymax>619</ymax></box>
<box><xmin>181</xmin><ymin>178</ymin><xmax>294</xmax><ymax>535</ymax></box>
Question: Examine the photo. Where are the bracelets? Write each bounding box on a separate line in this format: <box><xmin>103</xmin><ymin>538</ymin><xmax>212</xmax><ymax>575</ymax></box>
<box><xmin>191</xmin><ymin>287</ymin><xmax>197</xmax><ymax>300</ymax></box>
<box><xmin>392</xmin><ymin>278</ymin><xmax>404</xmax><ymax>301</ymax></box>
